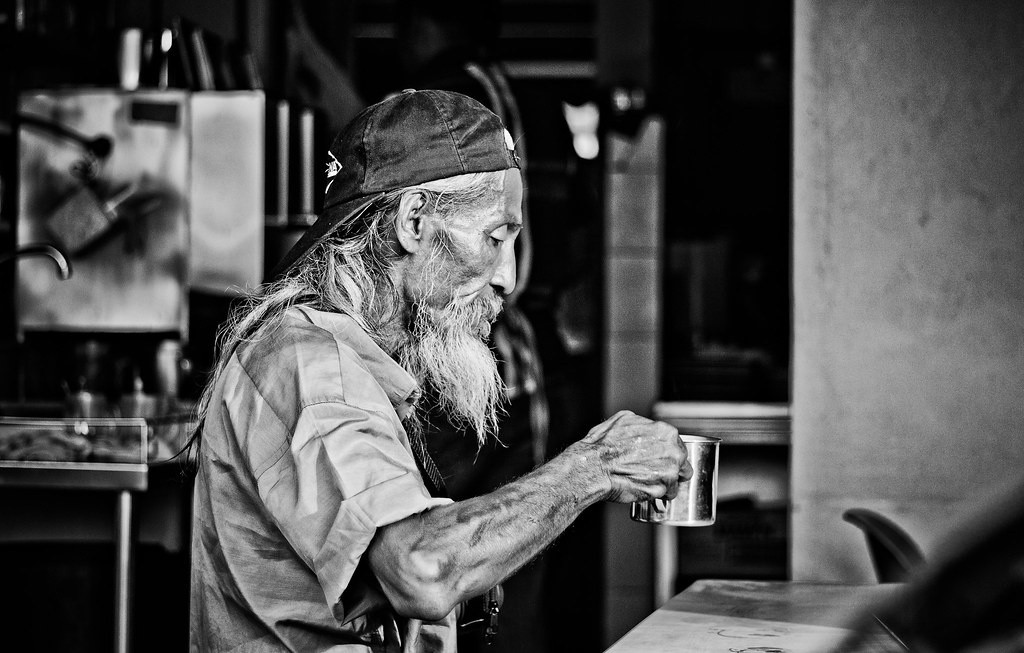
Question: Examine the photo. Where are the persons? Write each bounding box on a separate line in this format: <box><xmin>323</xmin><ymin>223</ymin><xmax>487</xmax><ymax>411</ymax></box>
<box><xmin>166</xmin><ymin>85</ymin><xmax>695</xmax><ymax>653</ymax></box>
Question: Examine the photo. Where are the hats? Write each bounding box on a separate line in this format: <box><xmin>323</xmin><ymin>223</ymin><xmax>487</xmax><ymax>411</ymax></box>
<box><xmin>262</xmin><ymin>88</ymin><xmax>521</xmax><ymax>284</ymax></box>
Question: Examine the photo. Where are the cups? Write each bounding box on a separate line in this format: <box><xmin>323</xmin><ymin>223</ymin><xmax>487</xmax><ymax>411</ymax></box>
<box><xmin>630</xmin><ymin>434</ymin><xmax>723</xmax><ymax>526</ymax></box>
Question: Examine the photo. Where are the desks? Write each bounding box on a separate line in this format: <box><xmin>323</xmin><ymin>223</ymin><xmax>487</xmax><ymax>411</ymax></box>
<box><xmin>647</xmin><ymin>401</ymin><xmax>791</xmax><ymax>610</ymax></box>
<box><xmin>604</xmin><ymin>577</ymin><xmax>904</xmax><ymax>653</ymax></box>
